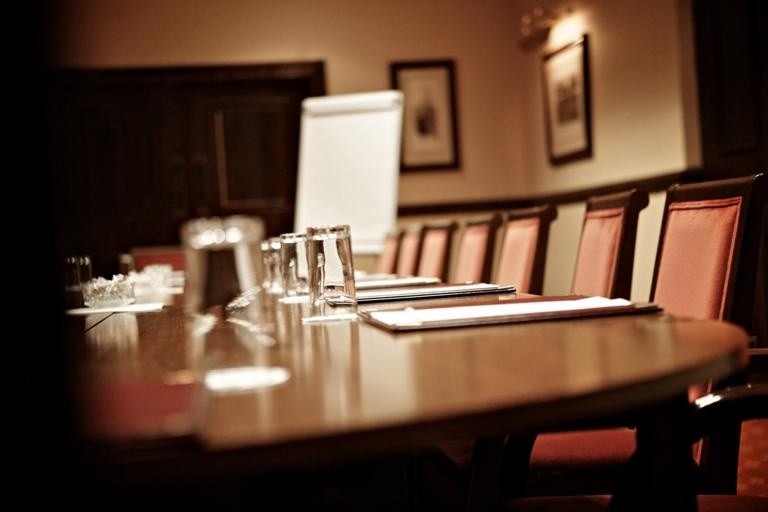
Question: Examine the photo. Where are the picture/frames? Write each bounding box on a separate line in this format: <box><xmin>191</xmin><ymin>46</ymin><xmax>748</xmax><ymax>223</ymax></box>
<box><xmin>390</xmin><ymin>59</ymin><xmax>460</xmax><ymax>169</ymax></box>
<box><xmin>540</xmin><ymin>34</ymin><xmax>593</xmax><ymax>164</ymax></box>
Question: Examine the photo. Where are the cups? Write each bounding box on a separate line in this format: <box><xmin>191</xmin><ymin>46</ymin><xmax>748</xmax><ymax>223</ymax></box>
<box><xmin>258</xmin><ymin>223</ymin><xmax>359</xmax><ymax>321</ymax></box>
<box><xmin>62</xmin><ymin>256</ymin><xmax>91</xmax><ymax>293</ymax></box>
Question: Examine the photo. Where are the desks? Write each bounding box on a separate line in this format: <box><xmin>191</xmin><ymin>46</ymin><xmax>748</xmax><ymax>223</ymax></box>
<box><xmin>80</xmin><ymin>265</ymin><xmax>752</xmax><ymax>511</ymax></box>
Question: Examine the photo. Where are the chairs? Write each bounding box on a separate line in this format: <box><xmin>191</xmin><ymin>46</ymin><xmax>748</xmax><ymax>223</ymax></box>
<box><xmin>507</xmin><ymin>172</ymin><xmax>760</xmax><ymax>498</ymax></box>
<box><xmin>490</xmin><ymin>201</ymin><xmax>561</xmax><ymax>295</ymax></box>
<box><xmin>373</xmin><ymin>218</ymin><xmax>457</xmax><ymax>285</ymax></box>
<box><xmin>451</xmin><ymin>213</ymin><xmax>503</xmax><ymax>290</ymax></box>
<box><xmin>562</xmin><ymin>184</ymin><xmax>651</xmax><ymax>308</ymax></box>
